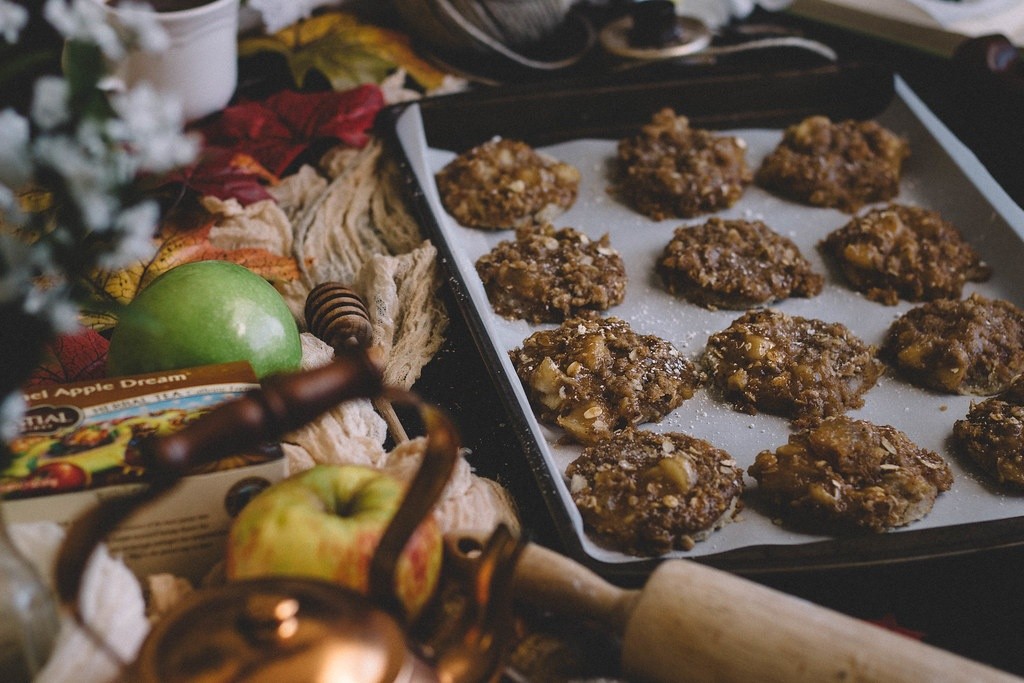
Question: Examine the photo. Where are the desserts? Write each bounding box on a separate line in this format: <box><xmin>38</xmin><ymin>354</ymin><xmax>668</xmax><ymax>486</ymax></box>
<box><xmin>431</xmin><ymin>103</ymin><xmax>1024</xmax><ymax>558</ymax></box>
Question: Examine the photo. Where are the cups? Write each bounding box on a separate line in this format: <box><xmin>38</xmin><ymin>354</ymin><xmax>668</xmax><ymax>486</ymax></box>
<box><xmin>94</xmin><ymin>0</ymin><xmax>239</xmax><ymax>123</ymax></box>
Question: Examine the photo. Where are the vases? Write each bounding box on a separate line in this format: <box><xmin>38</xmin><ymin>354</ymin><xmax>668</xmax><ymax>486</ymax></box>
<box><xmin>98</xmin><ymin>0</ymin><xmax>237</xmax><ymax>124</ymax></box>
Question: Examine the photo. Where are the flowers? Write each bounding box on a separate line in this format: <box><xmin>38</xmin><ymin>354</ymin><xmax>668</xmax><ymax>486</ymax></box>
<box><xmin>0</xmin><ymin>0</ymin><xmax>338</xmax><ymax>456</ymax></box>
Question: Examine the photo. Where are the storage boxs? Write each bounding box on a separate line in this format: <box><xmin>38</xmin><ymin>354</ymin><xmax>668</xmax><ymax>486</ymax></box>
<box><xmin>0</xmin><ymin>360</ymin><xmax>288</xmax><ymax>596</ymax></box>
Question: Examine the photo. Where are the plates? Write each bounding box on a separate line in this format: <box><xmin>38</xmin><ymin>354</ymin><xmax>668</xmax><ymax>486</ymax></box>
<box><xmin>376</xmin><ymin>57</ymin><xmax>1024</xmax><ymax>585</ymax></box>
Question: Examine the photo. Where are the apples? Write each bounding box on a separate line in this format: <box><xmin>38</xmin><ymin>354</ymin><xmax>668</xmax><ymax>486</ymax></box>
<box><xmin>226</xmin><ymin>462</ymin><xmax>442</xmax><ymax>629</ymax></box>
<box><xmin>105</xmin><ymin>257</ymin><xmax>302</xmax><ymax>386</ymax></box>
